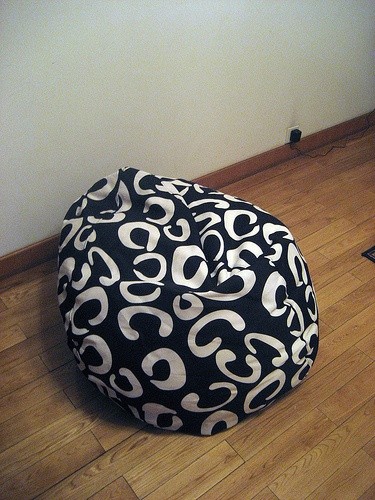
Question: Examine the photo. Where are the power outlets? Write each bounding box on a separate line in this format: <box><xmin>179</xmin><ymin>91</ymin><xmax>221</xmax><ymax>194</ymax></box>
<box><xmin>286</xmin><ymin>125</ymin><xmax>299</xmax><ymax>143</ymax></box>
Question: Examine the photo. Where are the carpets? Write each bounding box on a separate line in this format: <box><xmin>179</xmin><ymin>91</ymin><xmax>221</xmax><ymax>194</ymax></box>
<box><xmin>361</xmin><ymin>245</ymin><xmax>375</xmax><ymax>263</ymax></box>
